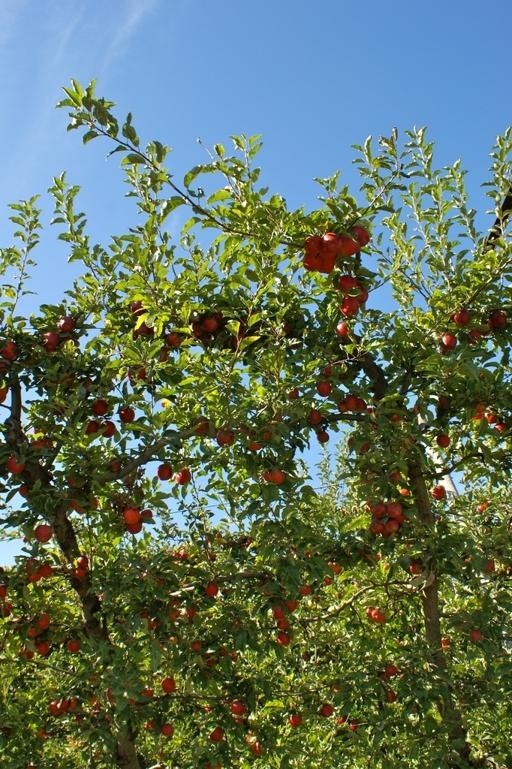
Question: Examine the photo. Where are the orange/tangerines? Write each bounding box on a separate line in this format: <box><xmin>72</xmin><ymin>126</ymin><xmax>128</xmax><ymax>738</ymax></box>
<box><xmin>0</xmin><ymin>272</ymin><xmax>506</xmax><ymax>754</ymax></box>
<box><xmin>303</xmin><ymin>227</ymin><xmax>369</xmax><ymax>271</ymax></box>
